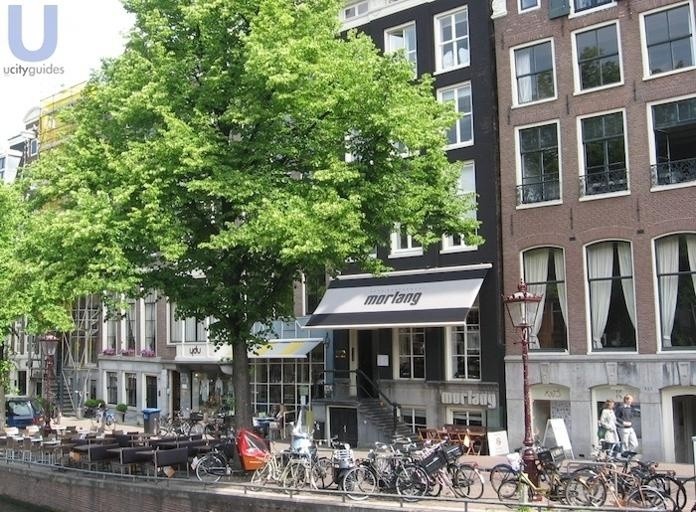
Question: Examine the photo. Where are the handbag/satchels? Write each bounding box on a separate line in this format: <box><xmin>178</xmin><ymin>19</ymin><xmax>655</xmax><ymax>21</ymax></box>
<box><xmin>598</xmin><ymin>427</ymin><xmax>606</xmax><ymax>438</ymax></box>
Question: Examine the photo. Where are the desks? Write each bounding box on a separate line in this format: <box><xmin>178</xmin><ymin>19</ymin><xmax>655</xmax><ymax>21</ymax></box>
<box><xmin>0</xmin><ymin>426</ymin><xmax>217</xmax><ymax>479</ymax></box>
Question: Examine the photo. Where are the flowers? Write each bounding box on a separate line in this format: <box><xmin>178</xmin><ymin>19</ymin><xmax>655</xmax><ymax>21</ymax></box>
<box><xmin>103</xmin><ymin>347</ymin><xmax>154</xmax><ymax>356</ymax></box>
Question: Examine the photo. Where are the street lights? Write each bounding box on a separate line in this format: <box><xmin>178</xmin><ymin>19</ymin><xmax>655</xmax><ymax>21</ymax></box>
<box><xmin>37</xmin><ymin>331</ymin><xmax>60</xmax><ymax>441</ymax></box>
<box><xmin>502</xmin><ymin>274</ymin><xmax>542</xmax><ymax>499</ymax></box>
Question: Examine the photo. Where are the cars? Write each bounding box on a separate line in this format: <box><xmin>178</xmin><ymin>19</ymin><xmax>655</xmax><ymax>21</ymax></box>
<box><xmin>6</xmin><ymin>393</ymin><xmax>49</xmax><ymax>431</ymax></box>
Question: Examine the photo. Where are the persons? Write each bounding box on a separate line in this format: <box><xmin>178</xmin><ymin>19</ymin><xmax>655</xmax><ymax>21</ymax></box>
<box><xmin>615</xmin><ymin>392</ymin><xmax>638</xmax><ymax>453</ymax></box>
<box><xmin>600</xmin><ymin>399</ymin><xmax>619</xmax><ymax>463</ymax></box>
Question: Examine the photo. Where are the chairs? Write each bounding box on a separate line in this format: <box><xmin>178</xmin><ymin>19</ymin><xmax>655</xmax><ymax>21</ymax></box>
<box><xmin>417</xmin><ymin>424</ymin><xmax>486</xmax><ymax>456</ymax></box>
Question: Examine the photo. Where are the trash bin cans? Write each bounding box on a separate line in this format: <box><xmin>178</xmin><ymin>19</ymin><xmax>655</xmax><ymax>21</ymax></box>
<box><xmin>257</xmin><ymin>418</ymin><xmax>274</xmax><ymax>437</ymax></box>
<box><xmin>141</xmin><ymin>408</ymin><xmax>161</xmax><ymax>435</ymax></box>
<box><xmin>313</xmin><ymin>421</ymin><xmax>325</xmax><ymax>439</ymax></box>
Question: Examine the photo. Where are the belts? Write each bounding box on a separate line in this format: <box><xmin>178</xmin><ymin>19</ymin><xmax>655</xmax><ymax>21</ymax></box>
<box><xmin>616</xmin><ymin>426</ymin><xmax>632</xmax><ymax>428</ymax></box>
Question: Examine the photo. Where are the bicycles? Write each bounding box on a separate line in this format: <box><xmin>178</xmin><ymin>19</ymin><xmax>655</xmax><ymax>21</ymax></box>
<box><xmin>88</xmin><ymin>402</ymin><xmax>118</xmax><ymax>433</ymax></box>
